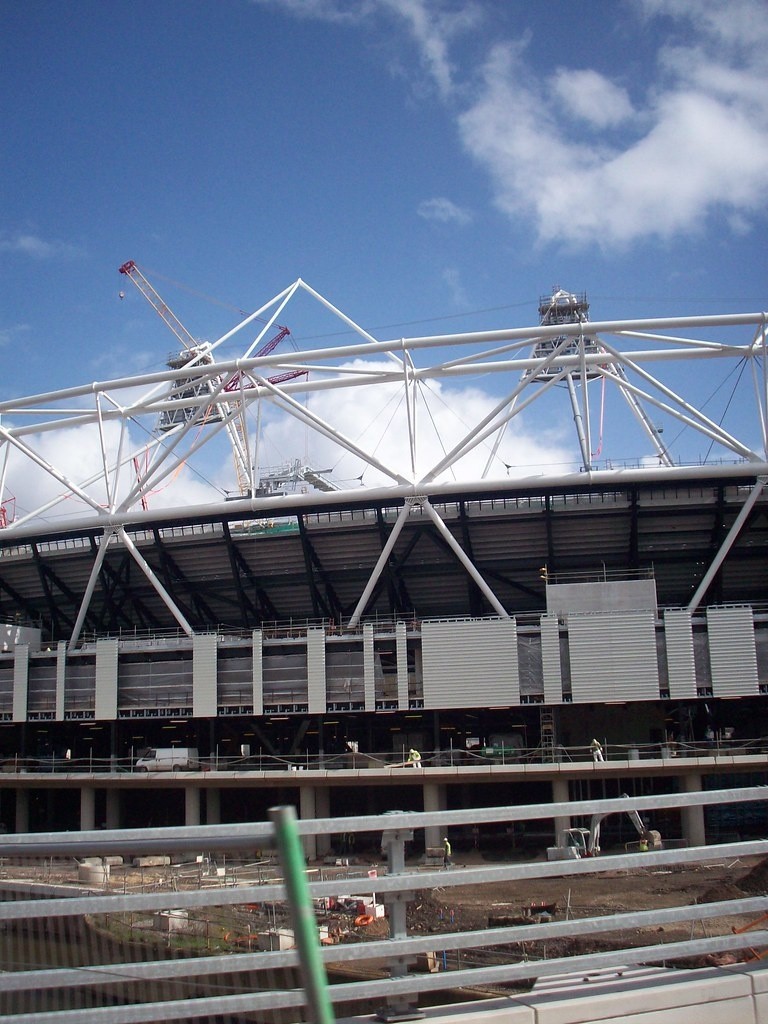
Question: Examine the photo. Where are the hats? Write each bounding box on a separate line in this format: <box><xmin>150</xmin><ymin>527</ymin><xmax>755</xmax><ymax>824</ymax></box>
<box><xmin>594</xmin><ymin>739</ymin><xmax>596</xmax><ymax>742</ymax></box>
<box><xmin>444</xmin><ymin>838</ymin><xmax>447</xmax><ymax>841</ymax></box>
<box><xmin>410</xmin><ymin>749</ymin><xmax>414</xmax><ymax>753</ymax></box>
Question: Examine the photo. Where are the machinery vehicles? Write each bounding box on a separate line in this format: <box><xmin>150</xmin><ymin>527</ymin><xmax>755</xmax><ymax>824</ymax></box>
<box><xmin>545</xmin><ymin>792</ymin><xmax>662</xmax><ymax>860</ymax></box>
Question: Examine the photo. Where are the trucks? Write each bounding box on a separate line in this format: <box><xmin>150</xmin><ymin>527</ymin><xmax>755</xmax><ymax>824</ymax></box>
<box><xmin>134</xmin><ymin>748</ymin><xmax>198</xmax><ymax>772</ymax></box>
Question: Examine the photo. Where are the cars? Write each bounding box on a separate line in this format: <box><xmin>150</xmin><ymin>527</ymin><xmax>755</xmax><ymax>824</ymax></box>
<box><xmin>380</xmin><ymin>842</ymin><xmax>406</xmax><ymax>860</ymax></box>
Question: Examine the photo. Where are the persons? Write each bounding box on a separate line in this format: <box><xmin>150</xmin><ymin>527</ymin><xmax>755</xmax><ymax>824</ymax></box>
<box><xmin>408</xmin><ymin>749</ymin><xmax>421</xmax><ymax>767</ymax></box>
<box><xmin>443</xmin><ymin>838</ymin><xmax>453</xmax><ymax>867</ymax></box>
<box><xmin>590</xmin><ymin>739</ymin><xmax>604</xmax><ymax>762</ymax></box>
<box><xmin>639</xmin><ymin>838</ymin><xmax>648</xmax><ymax>851</ymax></box>
<box><xmin>706</xmin><ymin>728</ymin><xmax>714</xmax><ymax>741</ymax></box>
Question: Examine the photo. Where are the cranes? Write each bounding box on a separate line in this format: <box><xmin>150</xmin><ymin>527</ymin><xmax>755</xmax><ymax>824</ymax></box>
<box><xmin>117</xmin><ymin>259</ymin><xmax>343</xmax><ymax>496</ymax></box>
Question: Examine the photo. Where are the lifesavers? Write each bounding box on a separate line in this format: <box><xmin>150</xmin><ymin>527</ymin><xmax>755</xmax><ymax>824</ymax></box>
<box><xmin>355</xmin><ymin>914</ymin><xmax>373</xmax><ymax>926</ymax></box>
